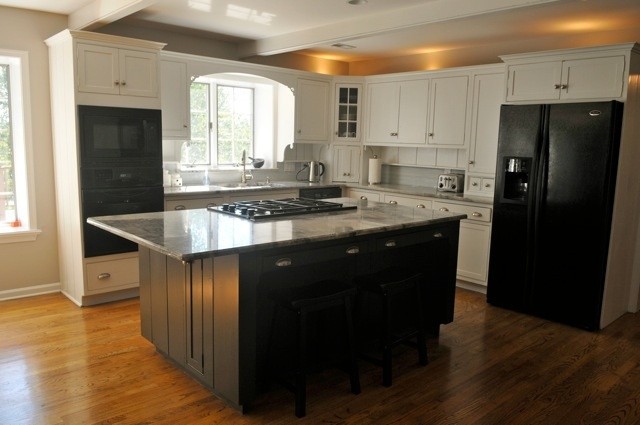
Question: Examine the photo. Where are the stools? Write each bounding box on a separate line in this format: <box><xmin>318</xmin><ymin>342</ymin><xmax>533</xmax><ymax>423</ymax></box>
<box><xmin>358</xmin><ymin>268</ymin><xmax>433</xmax><ymax>387</ymax></box>
<box><xmin>260</xmin><ymin>276</ymin><xmax>361</xmax><ymax>419</ymax></box>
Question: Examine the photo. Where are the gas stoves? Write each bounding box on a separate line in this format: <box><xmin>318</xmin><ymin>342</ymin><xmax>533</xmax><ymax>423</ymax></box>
<box><xmin>222</xmin><ymin>198</ymin><xmax>343</xmax><ymax>216</ymax></box>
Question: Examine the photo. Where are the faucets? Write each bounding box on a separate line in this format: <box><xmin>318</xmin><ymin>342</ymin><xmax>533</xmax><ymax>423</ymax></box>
<box><xmin>241</xmin><ymin>149</ymin><xmax>253</xmax><ymax>184</ymax></box>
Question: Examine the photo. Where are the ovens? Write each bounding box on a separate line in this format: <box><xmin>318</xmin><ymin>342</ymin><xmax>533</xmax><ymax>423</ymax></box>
<box><xmin>77</xmin><ymin>106</ymin><xmax>165</xmax><ymax>258</ymax></box>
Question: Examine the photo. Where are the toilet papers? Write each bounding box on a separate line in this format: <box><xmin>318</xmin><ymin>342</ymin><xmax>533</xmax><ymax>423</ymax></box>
<box><xmin>367</xmin><ymin>157</ymin><xmax>382</xmax><ymax>185</ymax></box>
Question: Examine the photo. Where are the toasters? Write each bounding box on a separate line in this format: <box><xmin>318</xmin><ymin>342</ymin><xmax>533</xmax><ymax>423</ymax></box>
<box><xmin>437</xmin><ymin>174</ymin><xmax>464</xmax><ymax>193</ymax></box>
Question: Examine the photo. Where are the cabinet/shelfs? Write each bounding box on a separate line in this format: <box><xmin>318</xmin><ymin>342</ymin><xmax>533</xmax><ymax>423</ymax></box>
<box><xmin>366</xmin><ymin>72</ymin><xmax>427</xmax><ymax>148</ymax></box>
<box><xmin>428</xmin><ymin>69</ymin><xmax>468</xmax><ymax>148</ymax></box>
<box><xmin>466</xmin><ymin>175</ymin><xmax>494</xmax><ymax>200</ymax></box>
<box><xmin>278</xmin><ymin>75</ymin><xmax>331</xmax><ymax>144</ymax></box>
<box><xmin>166</xmin><ymin>197</ymin><xmax>223</xmax><ymax>210</ymax></box>
<box><xmin>160</xmin><ymin>56</ymin><xmax>191</xmax><ymax>141</ymax></box>
<box><xmin>456</xmin><ymin>148</ymin><xmax>467</xmax><ymax>170</ymax></box>
<box><xmin>435</xmin><ymin>199</ymin><xmax>494</xmax><ymax>287</ymax></box>
<box><xmin>506</xmin><ymin>46</ymin><xmax>629</xmax><ymax>103</ymax></box>
<box><xmin>346</xmin><ymin>189</ymin><xmax>434</xmax><ymax>210</ymax></box>
<box><xmin>467</xmin><ymin>67</ymin><xmax>508</xmax><ymax>174</ymax></box>
<box><xmin>332</xmin><ymin>75</ymin><xmax>365</xmax><ymax>144</ymax></box>
<box><xmin>332</xmin><ymin>144</ymin><xmax>361</xmax><ymax>183</ymax></box>
<box><xmin>73</xmin><ymin>34</ymin><xmax>160</xmax><ymax>102</ymax></box>
<box><xmin>379</xmin><ymin>146</ymin><xmax>418</xmax><ymax>167</ymax></box>
<box><xmin>415</xmin><ymin>146</ymin><xmax>457</xmax><ymax>169</ymax></box>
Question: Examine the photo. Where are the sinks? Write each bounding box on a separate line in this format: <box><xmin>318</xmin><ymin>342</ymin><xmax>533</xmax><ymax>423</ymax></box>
<box><xmin>222</xmin><ymin>183</ymin><xmax>273</xmax><ymax>188</ymax></box>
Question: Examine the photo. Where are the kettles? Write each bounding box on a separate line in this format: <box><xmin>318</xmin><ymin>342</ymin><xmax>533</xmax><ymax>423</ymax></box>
<box><xmin>304</xmin><ymin>161</ymin><xmax>324</xmax><ymax>182</ymax></box>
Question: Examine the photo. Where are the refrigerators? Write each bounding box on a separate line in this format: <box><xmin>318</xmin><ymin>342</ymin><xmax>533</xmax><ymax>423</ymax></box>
<box><xmin>486</xmin><ymin>101</ymin><xmax>625</xmax><ymax>333</ymax></box>
